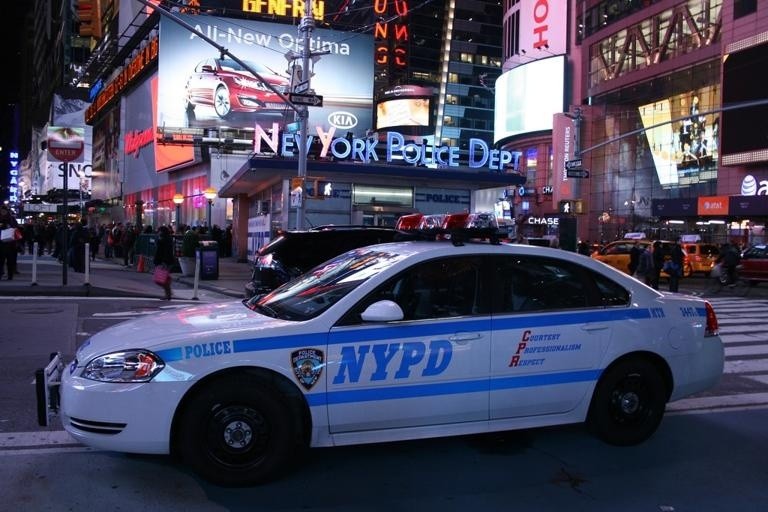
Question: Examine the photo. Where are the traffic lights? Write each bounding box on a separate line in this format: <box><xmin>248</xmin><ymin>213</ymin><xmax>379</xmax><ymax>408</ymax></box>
<box><xmin>558</xmin><ymin>201</ymin><xmax>570</xmax><ymax>214</ymax></box>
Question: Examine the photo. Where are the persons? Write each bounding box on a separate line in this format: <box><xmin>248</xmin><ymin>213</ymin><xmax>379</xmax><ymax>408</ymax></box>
<box><xmin>0</xmin><ymin>204</ymin><xmax>234</xmax><ymax>305</ymax></box>
<box><xmin>671</xmin><ymin>236</ymin><xmax>686</xmax><ymax>293</ymax></box>
<box><xmin>579</xmin><ymin>239</ymin><xmax>590</xmax><ymax>257</ymax></box>
<box><xmin>679</xmin><ymin>119</ymin><xmax>692</xmax><ymax>153</ymax></box>
<box><xmin>714</xmin><ymin>243</ymin><xmax>739</xmax><ymax>289</ymax></box>
<box><xmin>694</xmin><ymin>129</ymin><xmax>707</xmax><ymax>156</ymax></box>
<box><xmin>690</xmin><ymin>102</ymin><xmax>700</xmax><ymax>123</ymax></box>
<box><xmin>636</xmin><ymin>244</ymin><xmax>652</xmax><ymax>277</ymax></box>
<box><xmin>629</xmin><ymin>241</ymin><xmax>641</xmax><ymax>276</ymax></box>
<box><xmin>644</xmin><ymin>241</ymin><xmax>664</xmax><ymax>292</ymax></box>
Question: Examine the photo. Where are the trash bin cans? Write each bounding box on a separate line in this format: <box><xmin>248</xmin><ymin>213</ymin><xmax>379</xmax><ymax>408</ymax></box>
<box><xmin>200</xmin><ymin>246</ymin><xmax>219</xmax><ymax>280</ymax></box>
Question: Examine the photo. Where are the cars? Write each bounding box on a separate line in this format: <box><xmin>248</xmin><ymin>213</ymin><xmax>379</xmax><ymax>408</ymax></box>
<box><xmin>242</xmin><ymin>224</ymin><xmax>423</xmax><ymax>302</ymax></box>
<box><xmin>184</xmin><ymin>59</ymin><xmax>299</xmax><ymax>121</ymax></box>
<box><xmin>35</xmin><ymin>240</ymin><xmax>726</xmax><ymax>500</ymax></box>
<box><xmin>588</xmin><ymin>236</ymin><xmax>768</xmax><ymax>288</ymax></box>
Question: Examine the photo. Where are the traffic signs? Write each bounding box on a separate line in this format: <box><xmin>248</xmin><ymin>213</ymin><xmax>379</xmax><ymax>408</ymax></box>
<box><xmin>289</xmin><ymin>92</ymin><xmax>322</xmax><ymax>106</ymax></box>
<box><xmin>293</xmin><ymin>80</ymin><xmax>308</xmax><ymax>93</ymax></box>
<box><xmin>566</xmin><ymin>169</ymin><xmax>589</xmax><ymax>178</ymax></box>
<box><xmin>564</xmin><ymin>159</ymin><xmax>581</xmax><ymax>169</ymax></box>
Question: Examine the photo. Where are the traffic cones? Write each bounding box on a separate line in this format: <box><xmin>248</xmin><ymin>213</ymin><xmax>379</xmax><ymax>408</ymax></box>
<box><xmin>136</xmin><ymin>255</ymin><xmax>144</xmax><ymax>272</ymax></box>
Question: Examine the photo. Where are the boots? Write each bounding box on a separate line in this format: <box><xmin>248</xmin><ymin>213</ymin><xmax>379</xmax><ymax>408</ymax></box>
<box><xmin>158</xmin><ymin>291</ymin><xmax>172</xmax><ymax>300</ymax></box>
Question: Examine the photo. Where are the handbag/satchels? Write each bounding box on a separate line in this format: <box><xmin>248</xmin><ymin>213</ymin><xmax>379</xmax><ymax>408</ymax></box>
<box><xmin>152</xmin><ymin>266</ymin><xmax>170</xmax><ymax>285</ymax></box>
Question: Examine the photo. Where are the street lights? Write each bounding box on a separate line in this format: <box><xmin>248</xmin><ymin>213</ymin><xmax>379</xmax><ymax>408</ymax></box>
<box><xmin>172</xmin><ymin>194</ymin><xmax>184</xmax><ymax>234</ymax></box>
<box><xmin>204</xmin><ymin>188</ymin><xmax>216</xmax><ymax>233</ymax></box>
